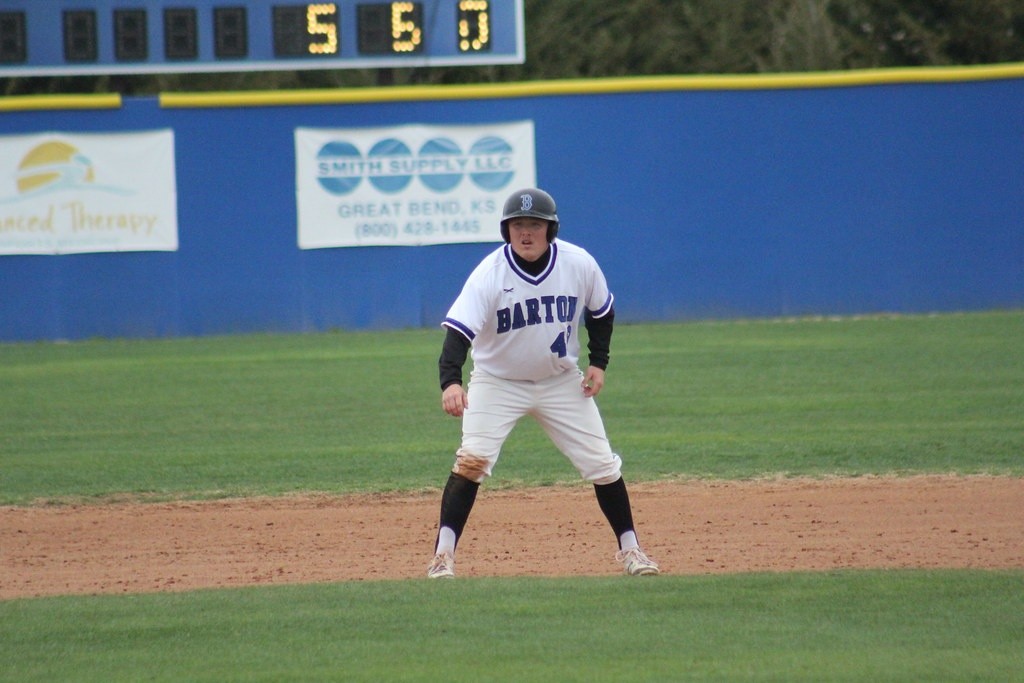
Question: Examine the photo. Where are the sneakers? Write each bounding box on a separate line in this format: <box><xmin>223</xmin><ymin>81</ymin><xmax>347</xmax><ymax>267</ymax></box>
<box><xmin>428</xmin><ymin>553</ymin><xmax>455</xmax><ymax>579</ymax></box>
<box><xmin>614</xmin><ymin>549</ymin><xmax>659</xmax><ymax>576</ymax></box>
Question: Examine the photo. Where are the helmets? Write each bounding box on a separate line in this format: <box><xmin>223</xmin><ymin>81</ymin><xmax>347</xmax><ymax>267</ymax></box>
<box><xmin>501</xmin><ymin>187</ymin><xmax>560</xmax><ymax>244</ymax></box>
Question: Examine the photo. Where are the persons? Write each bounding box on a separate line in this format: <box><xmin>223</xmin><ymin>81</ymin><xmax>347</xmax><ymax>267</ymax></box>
<box><xmin>427</xmin><ymin>188</ymin><xmax>660</xmax><ymax>575</ymax></box>
